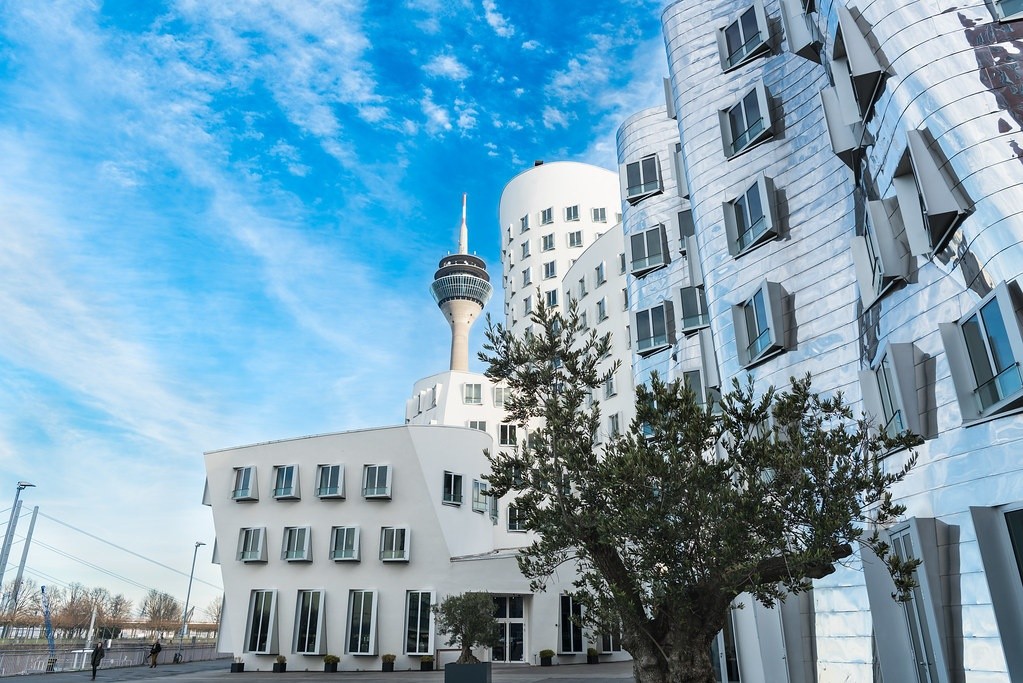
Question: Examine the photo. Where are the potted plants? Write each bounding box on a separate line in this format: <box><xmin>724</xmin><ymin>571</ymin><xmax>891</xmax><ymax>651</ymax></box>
<box><xmin>419</xmin><ymin>655</ymin><xmax>435</xmax><ymax>671</ymax></box>
<box><xmin>381</xmin><ymin>654</ymin><xmax>396</xmax><ymax>672</ymax></box>
<box><xmin>587</xmin><ymin>648</ymin><xmax>599</xmax><ymax>665</ymax></box>
<box><xmin>231</xmin><ymin>656</ymin><xmax>245</xmax><ymax>673</ymax></box>
<box><xmin>539</xmin><ymin>649</ymin><xmax>555</xmax><ymax>667</ymax></box>
<box><xmin>429</xmin><ymin>588</ymin><xmax>501</xmax><ymax>683</ymax></box>
<box><xmin>273</xmin><ymin>655</ymin><xmax>287</xmax><ymax>673</ymax></box>
<box><xmin>324</xmin><ymin>655</ymin><xmax>340</xmax><ymax>673</ymax></box>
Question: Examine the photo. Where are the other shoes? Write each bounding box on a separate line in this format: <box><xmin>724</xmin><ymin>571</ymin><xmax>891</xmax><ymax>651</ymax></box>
<box><xmin>154</xmin><ymin>665</ymin><xmax>156</xmax><ymax>668</ymax></box>
<box><xmin>91</xmin><ymin>678</ymin><xmax>95</xmax><ymax>681</ymax></box>
<box><xmin>149</xmin><ymin>666</ymin><xmax>152</xmax><ymax>668</ymax></box>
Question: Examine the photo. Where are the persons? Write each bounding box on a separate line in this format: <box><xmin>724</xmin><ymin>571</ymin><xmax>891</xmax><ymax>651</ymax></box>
<box><xmin>91</xmin><ymin>642</ymin><xmax>105</xmax><ymax>681</ymax></box>
<box><xmin>148</xmin><ymin>639</ymin><xmax>161</xmax><ymax>668</ymax></box>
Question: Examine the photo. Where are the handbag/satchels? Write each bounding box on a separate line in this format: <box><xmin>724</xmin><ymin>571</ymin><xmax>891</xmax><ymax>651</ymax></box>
<box><xmin>150</xmin><ymin>649</ymin><xmax>156</xmax><ymax>654</ymax></box>
